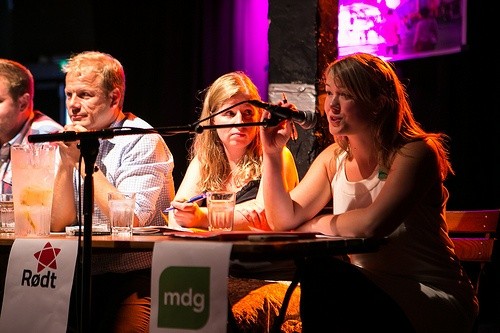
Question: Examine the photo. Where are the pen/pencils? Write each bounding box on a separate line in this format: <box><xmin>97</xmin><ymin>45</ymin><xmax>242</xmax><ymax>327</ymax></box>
<box><xmin>163</xmin><ymin>194</ymin><xmax>204</xmax><ymax>213</ymax></box>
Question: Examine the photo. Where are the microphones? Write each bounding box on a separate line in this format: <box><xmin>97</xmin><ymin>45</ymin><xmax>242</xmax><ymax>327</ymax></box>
<box><xmin>249</xmin><ymin>100</ymin><xmax>317</xmax><ymax>130</ymax></box>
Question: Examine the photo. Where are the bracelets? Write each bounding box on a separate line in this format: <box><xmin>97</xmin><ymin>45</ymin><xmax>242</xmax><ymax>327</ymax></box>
<box><xmin>81</xmin><ymin>164</ymin><xmax>99</xmax><ymax>180</ymax></box>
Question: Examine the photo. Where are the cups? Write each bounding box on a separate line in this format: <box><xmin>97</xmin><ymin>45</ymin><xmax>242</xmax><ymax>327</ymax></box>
<box><xmin>206</xmin><ymin>192</ymin><xmax>236</xmax><ymax>231</ymax></box>
<box><xmin>0</xmin><ymin>194</ymin><xmax>16</xmax><ymax>233</ymax></box>
<box><xmin>108</xmin><ymin>199</ymin><xmax>135</xmax><ymax>238</ymax></box>
<box><xmin>10</xmin><ymin>143</ymin><xmax>57</xmax><ymax>237</ymax></box>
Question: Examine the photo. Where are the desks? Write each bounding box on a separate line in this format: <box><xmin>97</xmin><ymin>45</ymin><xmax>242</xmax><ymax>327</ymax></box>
<box><xmin>0</xmin><ymin>234</ymin><xmax>367</xmax><ymax>332</ymax></box>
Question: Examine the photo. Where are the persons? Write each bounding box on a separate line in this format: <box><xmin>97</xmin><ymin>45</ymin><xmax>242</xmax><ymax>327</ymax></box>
<box><xmin>50</xmin><ymin>52</ymin><xmax>175</xmax><ymax>333</ymax></box>
<box><xmin>261</xmin><ymin>52</ymin><xmax>478</xmax><ymax>333</ymax></box>
<box><xmin>0</xmin><ymin>60</ymin><xmax>64</xmax><ymax>195</ymax></box>
<box><xmin>169</xmin><ymin>72</ymin><xmax>299</xmax><ymax>333</ymax></box>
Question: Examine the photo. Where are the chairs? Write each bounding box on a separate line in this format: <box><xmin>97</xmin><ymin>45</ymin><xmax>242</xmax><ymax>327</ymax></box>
<box><xmin>443</xmin><ymin>210</ymin><xmax>500</xmax><ymax>306</ymax></box>
